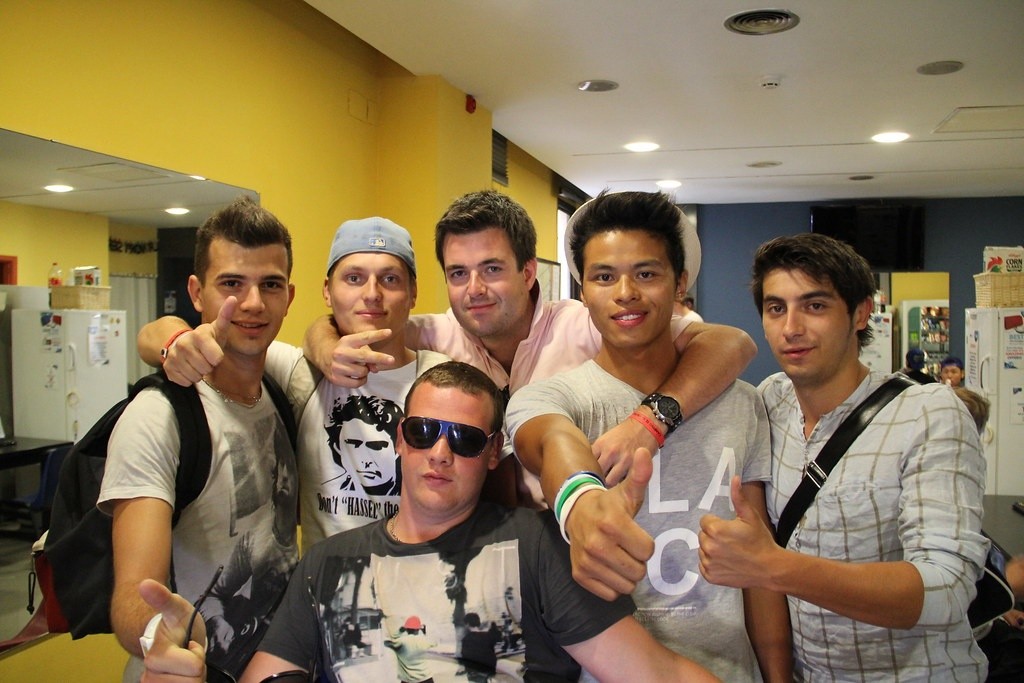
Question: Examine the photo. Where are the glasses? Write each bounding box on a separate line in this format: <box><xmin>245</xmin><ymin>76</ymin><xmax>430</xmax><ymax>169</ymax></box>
<box><xmin>401</xmin><ymin>418</ymin><xmax>497</xmax><ymax>459</ymax></box>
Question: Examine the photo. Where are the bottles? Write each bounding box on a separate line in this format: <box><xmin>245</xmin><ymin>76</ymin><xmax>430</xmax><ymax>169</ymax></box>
<box><xmin>48</xmin><ymin>262</ymin><xmax>64</xmax><ymax>308</ymax></box>
<box><xmin>874</xmin><ymin>290</ymin><xmax>886</xmax><ymax>313</ymax></box>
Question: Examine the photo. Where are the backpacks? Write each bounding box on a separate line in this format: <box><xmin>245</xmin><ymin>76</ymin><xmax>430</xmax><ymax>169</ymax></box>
<box><xmin>26</xmin><ymin>361</ymin><xmax>299</xmax><ymax>641</ymax></box>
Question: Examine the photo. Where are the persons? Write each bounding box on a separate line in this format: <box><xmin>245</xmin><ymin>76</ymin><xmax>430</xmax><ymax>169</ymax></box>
<box><xmin>136</xmin><ymin>216</ymin><xmax>517</xmax><ymax>559</ymax></box>
<box><xmin>698</xmin><ymin>232</ymin><xmax>990</xmax><ymax>683</ymax></box>
<box><xmin>97</xmin><ymin>194</ymin><xmax>299</xmax><ymax>683</ymax></box>
<box><xmin>302</xmin><ymin>187</ymin><xmax>758</xmax><ymax>490</ymax></box>
<box><xmin>903</xmin><ymin>348</ymin><xmax>938</xmax><ymax>384</ymax></box>
<box><xmin>940</xmin><ymin>357</ymin><xmax>965</xmax><ymax>387</ymax></box>
<box><xmin>247</xmin><ymin>360</ymin><xmax>724</xmax><ymax>683</ymax></box>
<box><xmin>672</xmin><ymin>302</ymin><xmax>704</xmax><ymax>323</ymax></box>
<box><xmin>952</xmin><ymin>387</ymin><xmax>1024</xmax><ymax>683</ymax></box>
<box><xmin>503</xmin><ymin>186</ymin><xmax>792</xmax><ymax>683</ymax></box>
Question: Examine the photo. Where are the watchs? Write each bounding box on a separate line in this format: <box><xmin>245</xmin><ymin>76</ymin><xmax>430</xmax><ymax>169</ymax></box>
<box><xmin>139</xmin><ymin>613</ymin><xmax>163</xmax><ymax>658</ymax></box>
<box><xmin>640</xmin><ymin>393</ymin><xmax>683</xmax><ymax>434</ymax></box>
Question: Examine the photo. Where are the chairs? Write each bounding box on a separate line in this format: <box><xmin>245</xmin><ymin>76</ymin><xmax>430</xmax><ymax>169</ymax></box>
<box><xmin>0</xmin><ymin>445</ymin><xmax>73</xmax><ymax>539</ymax></box>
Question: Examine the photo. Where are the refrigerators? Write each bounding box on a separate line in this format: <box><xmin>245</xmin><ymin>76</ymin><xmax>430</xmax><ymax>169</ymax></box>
<box><xmin>12</xmin><ymin>309</ymin><xmax>128</xmax><ymax>443</ymax></box>
<box><xmin>965</xmin><ymin>307</ymin><xmax>1024</xmax><ymax>497</ymax></box>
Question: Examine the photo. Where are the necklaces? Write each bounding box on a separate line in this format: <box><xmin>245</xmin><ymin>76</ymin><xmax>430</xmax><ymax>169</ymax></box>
<box><xmin>390</xmin><ymin>510</ymin><xmax>401</xmax><ymax>540</ymax></box>
<box><xmin>201</xmin><ymin>377</ymin><xmax>260</xmax><ymax>407</ymax></box>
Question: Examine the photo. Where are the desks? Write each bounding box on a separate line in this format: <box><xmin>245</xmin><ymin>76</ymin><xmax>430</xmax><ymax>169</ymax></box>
<box><xmin>980</xmin><ymin>495</ymin><xmax>1024</xmax><ymax>561</ymax></box>
<box><xmin>0</xmin><ymin>436</ymin><xmax>74</xmax><ymax>479</ymax></box>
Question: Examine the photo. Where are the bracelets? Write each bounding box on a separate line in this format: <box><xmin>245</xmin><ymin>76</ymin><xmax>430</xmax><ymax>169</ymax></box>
<box><xmin>554</xmin><ymin>470</ymin><xmax>608</xmax><ymax>545</ymax></box>
<box><xmin>160</xmin><ymin>329</ymin><xmax>194</xmax><ymax>362</ymax></box>
<box><xmin>629</xmin><ymin>411</ymin><xmax>665</xmax><ymax>446</ymax></box>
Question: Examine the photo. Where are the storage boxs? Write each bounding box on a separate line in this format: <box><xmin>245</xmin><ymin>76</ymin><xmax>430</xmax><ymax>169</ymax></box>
<box><xmin>74</xmin><ymin>266</ymin><xmax>101</xmax><ymax>284</ymax></box>
<box><xmin>982</xmin><ymin>245</ymin><xmax>1024</xmax><ymax>272</ymax></box>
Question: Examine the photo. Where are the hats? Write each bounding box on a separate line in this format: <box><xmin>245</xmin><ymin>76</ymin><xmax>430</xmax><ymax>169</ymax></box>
<box><xmin>563</xmin><ymin>191</ymin><xmax>702</xmax><ymax>296</ymax></box>
<box><xmin>326</xmin><ymin>216</ymin><xmax>417</xmax><ymax>279</ymax></box>
